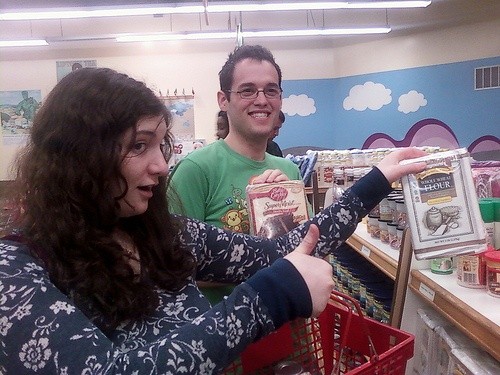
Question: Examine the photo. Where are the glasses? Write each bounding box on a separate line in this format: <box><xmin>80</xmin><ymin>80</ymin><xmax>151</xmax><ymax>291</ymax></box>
<box><xmin>229</xmin><ymin>87</ymin><xmax>283</xmax><ymax>98</ymax></box>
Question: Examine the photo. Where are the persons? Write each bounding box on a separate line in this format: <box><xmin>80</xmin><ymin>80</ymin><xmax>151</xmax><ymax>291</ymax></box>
<box><xmin>266</xmin><ymin>109</ymin><xmax>285</xmax><ymax>157</ymax></box>
<box><xmin>159</xmin><ymin>45</ymin><xmax>316</xmax><ymax>304</ymax></box>
<box><xmin>0</xmin><ymin>67</ymin><xmax>427</xmax><ymax>375</ymax></box>
<box><xmin>216</xmin><ymin>110</ymin><xmax>229</xmax><ymax>139</ymax></box>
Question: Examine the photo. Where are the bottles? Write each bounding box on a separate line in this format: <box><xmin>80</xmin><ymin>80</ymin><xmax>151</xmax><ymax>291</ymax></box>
<box><xmin>306</xmin><ymin>148</ymin><xmax>473</xmax><ymax>251</ymax></box>
<box><xmin>478</xmin><ymin>197</ymin><xmax>500</xmax><ymax>251</ymax></box>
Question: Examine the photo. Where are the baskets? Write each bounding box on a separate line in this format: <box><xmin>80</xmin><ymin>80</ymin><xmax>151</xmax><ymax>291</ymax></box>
<box><xmin>214</xmin><ymin>285</ymin><xmax>415</xmax><ymax>375</ymax></box>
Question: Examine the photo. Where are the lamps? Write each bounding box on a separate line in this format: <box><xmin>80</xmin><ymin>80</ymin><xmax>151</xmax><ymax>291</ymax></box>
<box><xmin>0</xmin><ymin>0</ymin><xmax>432</xmax><ymax>47</ymax></box>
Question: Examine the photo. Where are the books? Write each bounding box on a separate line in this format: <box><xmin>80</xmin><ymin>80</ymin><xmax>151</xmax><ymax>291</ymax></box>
<box><xmin>246</xmin><ymin>181</ymin><xmax>308</xmax><ymax>240</ymax></box>
